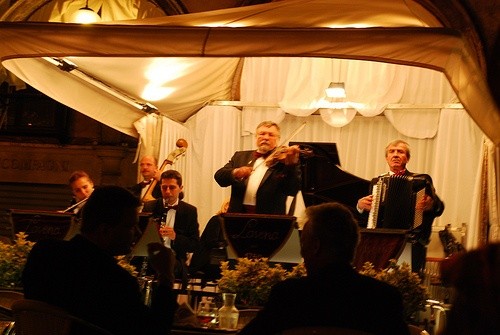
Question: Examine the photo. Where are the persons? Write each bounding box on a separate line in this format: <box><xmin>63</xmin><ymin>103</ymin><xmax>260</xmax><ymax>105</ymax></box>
<box><xmin>437</xmin><ymin>241</ymin><xmax>500</xmax><ymax>335</ymax></box>
<box><xmin>56</xmin><ymin>172</ymin><xmax>95</xmax><ymax>211</ymax></box>
<box><xmin>236</xmin><ymin>202</ymin><xmax>409</xmax><ymax>335</ymax></box>
<box><xmin>214</xmin><ymin>122</ymin><xmax>302</xmax><ymax>215</ymax></box>
<box><xmin>21</xmin><ymin>185</ymin><xmax>175</xmax><ymax>335</ymax></box>
<box><xmin>351</xmin><ymin>140</ymin><xmax>444</xmax><ymax>243</ymax></box>
<box><xmin>141</xmin><ymin>170</ymin><xmax>200</xmax><ymax>262</ymax></box>
<box><xmin>441</xmin><ymin>224</ymin><xmax>465</xmax><ymax>254</ymax></box>
<box><xmin>126</xmin><ymin>156</ymin><xmax>184</xmax><ymax>199</ymax></box>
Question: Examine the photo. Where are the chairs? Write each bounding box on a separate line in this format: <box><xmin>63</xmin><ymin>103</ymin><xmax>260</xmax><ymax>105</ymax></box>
<box><xmin>12</xmin><ymin>300</ymin><xmax>109</xmax><ymax>335</ymax></box>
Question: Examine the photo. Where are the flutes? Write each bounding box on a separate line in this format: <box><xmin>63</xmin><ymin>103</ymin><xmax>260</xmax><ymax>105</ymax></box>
<box><xmin>161</xmin><ymin>196</ymin><xmax>169</xmax><ymax>228</ymax></box>
<box><xmin>62</xmin><ymin>196</ymin><xmax>90</xmax><ymax>213</ymax></box>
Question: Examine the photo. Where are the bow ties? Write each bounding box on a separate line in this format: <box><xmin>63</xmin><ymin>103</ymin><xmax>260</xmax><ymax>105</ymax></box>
<box><xmin>255</xmin><ymin>152</ymin><xmax>269</xmax><ymax>159</ymax></box>
<box><xmin>164</xmin><ymin>205</ymin><xmax>179</xmax><ymax>211</ymax></box>
<box><xmin>142</xmin><ymin>180</ymin><xmax>151</xmax><ymax>187</ymax></box>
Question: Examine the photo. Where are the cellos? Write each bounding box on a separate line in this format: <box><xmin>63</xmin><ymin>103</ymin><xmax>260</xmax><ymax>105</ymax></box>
<box><xmin>138</xmin><ymin>138</ymin><xmax>188</xmax><ymax>212</ymax></box>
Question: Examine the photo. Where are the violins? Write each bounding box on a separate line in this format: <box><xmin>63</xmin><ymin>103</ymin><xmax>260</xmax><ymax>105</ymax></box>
<box><xmin>266</xmin><ymin>146</ymin><xmax>313</xmax><ymax>168</ymax></box>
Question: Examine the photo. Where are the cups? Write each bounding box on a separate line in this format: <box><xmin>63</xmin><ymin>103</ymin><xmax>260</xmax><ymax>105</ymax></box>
<box><xmin>195</xmin><ymin>302</ymin><xmax>216</xmax><ymax>326</ymax></box>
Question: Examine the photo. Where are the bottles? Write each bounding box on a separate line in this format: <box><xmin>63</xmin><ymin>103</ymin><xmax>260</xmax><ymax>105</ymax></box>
<box><xmin>218</xmin><ymin>293</ymin><xmax>239</xmax><ymax>330</ymax></box>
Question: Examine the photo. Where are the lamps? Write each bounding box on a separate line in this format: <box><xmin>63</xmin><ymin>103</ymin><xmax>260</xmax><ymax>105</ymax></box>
<box><xmin>72</xmin><ymin>0</ymin><xmax>101</xmax><ymax>24</ymax></box>
<box><xmin>327</xmin><ymin>58</ymin><xmax>346</xmax><ymax>98</ymax></box>
